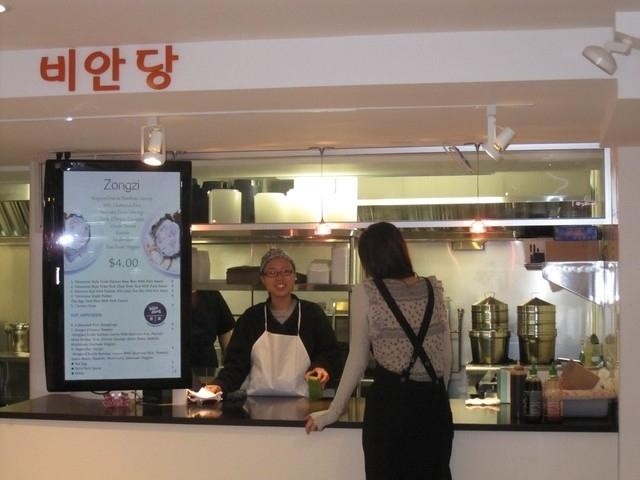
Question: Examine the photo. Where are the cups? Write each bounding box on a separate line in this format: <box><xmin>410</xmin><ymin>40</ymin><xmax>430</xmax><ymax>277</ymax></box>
<box><xmin>308</xmin><ymin>376</ymin><xmax>323</xmax><ymax>401</ymax></box>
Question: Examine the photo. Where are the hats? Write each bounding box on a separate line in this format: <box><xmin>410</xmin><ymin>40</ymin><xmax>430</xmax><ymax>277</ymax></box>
<box><xmin>259</xmin><ymin>247</ymin><xmax>295</xmax><ymax>274</ymax></box>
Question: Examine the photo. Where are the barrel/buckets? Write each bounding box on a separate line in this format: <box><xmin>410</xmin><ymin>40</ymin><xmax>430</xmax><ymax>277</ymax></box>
<box><xmin>4</xmin><ymin>325</ymin><xmax>28</xmax><ymax>353</ymax></box>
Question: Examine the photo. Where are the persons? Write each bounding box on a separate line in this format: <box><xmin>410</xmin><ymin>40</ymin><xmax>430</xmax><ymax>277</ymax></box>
<box><xmin>304</xmin><ymin>222</ymin><xmax>453</xmax><ymax>480</ymax></box>
<box><xmin>191</xmin><ymin>290</ymin><xmax>236</xmax><ymax>376</ymax></box>
<box><xmin>205</xmin><ymin>249</ymin><xmax>337</xmax><ymax>396</ymax></box>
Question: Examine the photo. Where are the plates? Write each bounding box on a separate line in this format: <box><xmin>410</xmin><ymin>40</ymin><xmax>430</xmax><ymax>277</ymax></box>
<box><xmin>140</xmin><ymin>207</ymin><xmax>181</xmax><ymax>275</ymax></box>
<box><xmin>64</xmin><ymin>200</ymin><xmax>105</xmax><ymax>273</ymax></box>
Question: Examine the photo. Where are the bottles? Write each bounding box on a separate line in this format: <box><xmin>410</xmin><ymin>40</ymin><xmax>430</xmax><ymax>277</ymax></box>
<box><xmin>584</xmin><ymin>333</ymin><xmax>601</xmax><ymax>366</ymax></box>
<box><xmin>510</xmin><ymin>359</ymin><xmax>563</xmax><ymax>424</ymax></box>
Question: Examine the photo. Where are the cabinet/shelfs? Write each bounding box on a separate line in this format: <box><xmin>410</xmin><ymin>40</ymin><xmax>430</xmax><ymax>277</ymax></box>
<box><xmin>189</xmin><ymin>231</ymin><xmax>357</xmax><ymax>294</ymax></box>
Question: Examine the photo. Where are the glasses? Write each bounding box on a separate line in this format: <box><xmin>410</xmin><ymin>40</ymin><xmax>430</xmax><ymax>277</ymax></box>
<box><xmin>263</xmin><ymin>269</ymin><xmax>294</xmax><ymax>278</ymax></box>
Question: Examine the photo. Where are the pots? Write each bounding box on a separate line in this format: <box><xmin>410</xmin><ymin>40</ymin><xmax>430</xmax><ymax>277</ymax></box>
<box><xmin>518</xmin><ymin>336</ymin><xmax>556</xmax><ymax>365</ymax></box>
<box><xmin>468</xmin><ymin>331</ymin><xmax>511</xmax><ymax>363</ymax></box>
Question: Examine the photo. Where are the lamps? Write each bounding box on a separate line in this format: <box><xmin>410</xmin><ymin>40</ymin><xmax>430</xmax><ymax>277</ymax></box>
<box><xmin>478</xmin><ymin>103</ymin><xmax>517</xmax><ymax>163</ymax></box>
<box><xmin>139</xmin><ymin>114</ymin><xmax>168</xmax><ymax>168</ymax></box>
<box><xmin>581</xmin><ymin>39</ymin><xmax>632</xmax><ymax>76</ymax></box>
